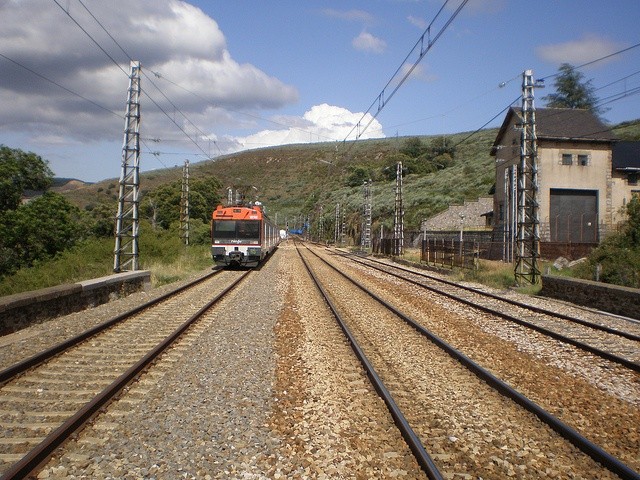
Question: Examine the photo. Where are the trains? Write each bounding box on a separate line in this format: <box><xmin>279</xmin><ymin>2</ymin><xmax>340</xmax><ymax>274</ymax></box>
<box><xmin>211</xmin><ymin>204</ymin><xmax>281</xmax><ymax>270</ymax></box>
